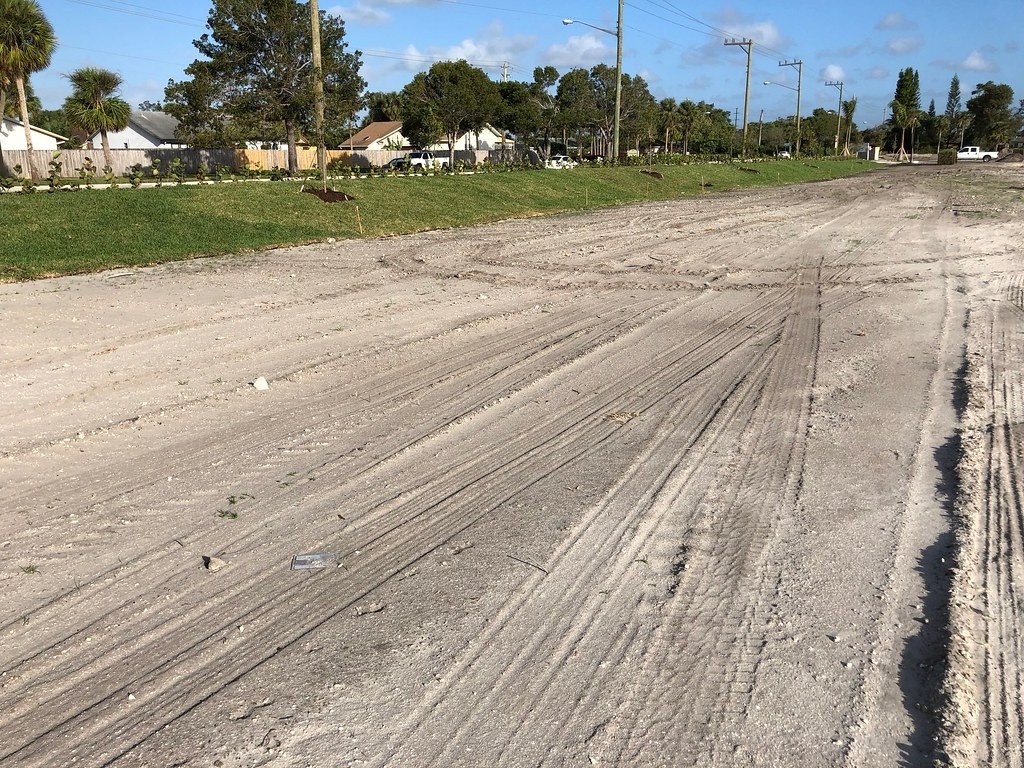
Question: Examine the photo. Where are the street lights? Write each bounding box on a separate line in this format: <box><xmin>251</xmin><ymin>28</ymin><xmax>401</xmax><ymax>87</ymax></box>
<box><xmin>562</xmin><ymin>18</ymin><xmax>623</xmax><ymax>159</ymax></box>
<box><xmin>827</xmin><ymin>111</ymin><xmax>841</xmax><ymax>156</ymax></box>
<box><xmin>763</xmin><ymin>81</ymin><xmax>802</xmax><ymax>160</ymax></box>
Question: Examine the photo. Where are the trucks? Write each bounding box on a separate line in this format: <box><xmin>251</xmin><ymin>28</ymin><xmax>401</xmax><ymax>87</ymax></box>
<box><xmin>405</xmin><ymin>151</ymin><xmax>450</xmax><ymax>175</ymax></box>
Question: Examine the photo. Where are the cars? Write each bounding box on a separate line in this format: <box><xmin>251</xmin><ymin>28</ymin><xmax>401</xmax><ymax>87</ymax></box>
<box><xmin>382</xmin><ymin>157</ymin><xmax>404</xmax><ymax>171</ymax></box>
<box><xmin>544</xmin><ymin>154</ymin><xmax>578</xmax><ymax>170</ymax></box>
<box><xmin>778</xmin><ymin>150</ymin><xmax>790</xmax><ymax>160</ymax></box>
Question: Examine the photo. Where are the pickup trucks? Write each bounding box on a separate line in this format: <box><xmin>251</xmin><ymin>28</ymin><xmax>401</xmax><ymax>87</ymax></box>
<box><xmin>957</xmin><ymin>146</ymin><xmax>999</xmax><ymax>162</ymax></box>
